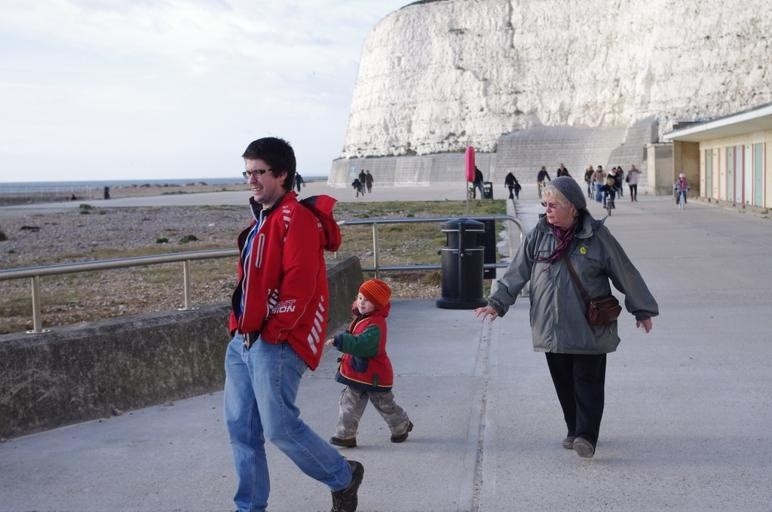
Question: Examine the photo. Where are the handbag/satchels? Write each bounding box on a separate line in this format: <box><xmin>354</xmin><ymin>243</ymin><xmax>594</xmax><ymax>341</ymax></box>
<box><xmin>588</xmin><ymin>295</ymin><xmax>622</xmax><ymax>324</ymax></box>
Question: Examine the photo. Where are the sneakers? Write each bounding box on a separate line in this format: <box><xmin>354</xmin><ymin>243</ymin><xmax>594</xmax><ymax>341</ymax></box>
<box><xmin>331</xmin><ymin>437</ymin><xmax>357</xmax><ymax>447</ymax></box>
<box><xmin>562</xmin><ymin>435</ymin><xmax>594</xmax><ymax>458</ymax></box>
<box><xmin>391</xmin><ymin>421</ymin><xmax>413</xmax><ymax>442</ymax></box>
<box><xmin>331</xmin><ymin>460</ymin><xmax>364</xmax><ymax>511</ymax></box>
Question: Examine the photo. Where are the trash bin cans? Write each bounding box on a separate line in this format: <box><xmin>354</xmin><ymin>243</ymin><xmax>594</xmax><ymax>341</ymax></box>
<box><xmin>436</xmin><ymin>218</ymin><xmax>489</xmax><ymax>309</ymax></box>
<box><xmin>482</xmin><ymin>182</ymin><xmax>493</xmax><ymax>199</ymax></box>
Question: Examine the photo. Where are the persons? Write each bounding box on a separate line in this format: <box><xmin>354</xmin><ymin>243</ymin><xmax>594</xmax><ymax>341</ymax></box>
<box><xmin>104</xmin><ymin>186</ymin><xmax>110</xmax><ymax>198</ymax></box>
<box><xmin>324</xmin><ymin>277</ymin><xmax>414</xmax><ymax>449</ymax></box>
<box><xmin>295</xmin><ymin>170</ymin><xmax>303</xmax><ymax>192</ymax></box>
<box><xmin>71</xmin><ymin>193</ymin><xmax>77</xmax><ymax>199</ymax></box>
<box><xmin>475</xmin><ymin>162</ymin><xmax>691</xmax><ymax>209</ymax></box>
<box><xmin>351</xmin><ymin>168</ymin><xmax>374</xmax><ymax>197</ymax></box>
<box><xmin>222</xmin><ymin>136</ymin><xmax>365</xmax><ymax>511</ymax></box>
<box><xmin>470</xmin><ymin>176</ymin><xmax>660</xmax><ymax>457</ymax></box>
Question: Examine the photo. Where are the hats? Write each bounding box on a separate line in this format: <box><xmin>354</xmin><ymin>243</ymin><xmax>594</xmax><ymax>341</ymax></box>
<box><xmin>359</xmin><ymin>280</ymin><xmax>392</xmax><ymax>309</ymax></box>
<box><xmin>550</xmin><ymin>176</ymin><xmax>586</xmax><ymax>209</ymax></box>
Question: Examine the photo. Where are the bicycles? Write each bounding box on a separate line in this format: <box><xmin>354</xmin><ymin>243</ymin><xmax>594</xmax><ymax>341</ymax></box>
<box><xmin>676</xmin><ymin>190</ymin><xmax>688</xmax><ymax>211</ymax></box>
<box><xmin>606</xmin><ymin>193</ymin><xmax>613</xmax><ymax>216</ymax></box>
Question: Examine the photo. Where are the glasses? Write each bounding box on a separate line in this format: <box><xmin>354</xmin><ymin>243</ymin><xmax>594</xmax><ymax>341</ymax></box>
<box><xmin>242</xmin><ymin>167</ymin><xmax>272</xmax><ymax>179</ymax></box>
<box><xmin>541</xmin><ymin>200</ymin><xmax>559</xmax><ymax>208</ymax></box>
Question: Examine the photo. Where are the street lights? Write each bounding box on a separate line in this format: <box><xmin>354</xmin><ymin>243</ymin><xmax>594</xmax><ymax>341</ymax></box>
<box><xmin>465</xmin><ymin>144</ymin><xmax>477</xmax><ymax>217</ymax></box>
